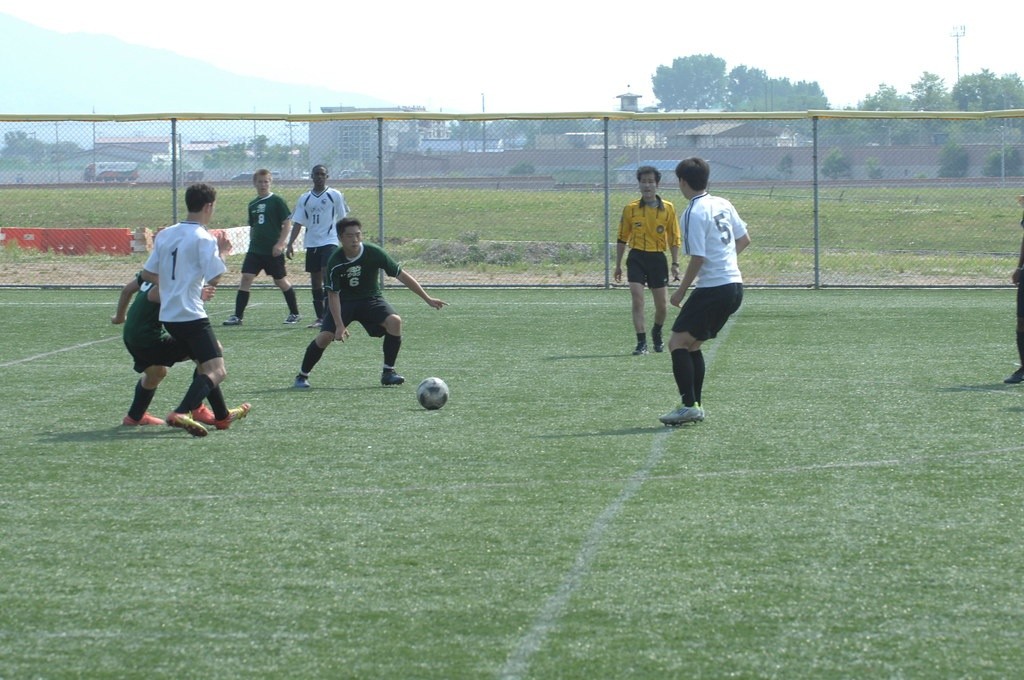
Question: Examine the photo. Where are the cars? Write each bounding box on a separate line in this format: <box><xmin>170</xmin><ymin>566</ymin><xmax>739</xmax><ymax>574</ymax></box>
<box><xmin>232</xmin><ymin>173</ymin><xmax>252</xmax><ymax>180</ymax></box>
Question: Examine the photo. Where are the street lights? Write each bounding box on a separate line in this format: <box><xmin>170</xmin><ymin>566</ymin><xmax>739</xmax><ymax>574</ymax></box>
<box><xmin>175</xmin><ymin>132</ymin><xmax>183</xmax><ymax>187</ymax></box>
<box><xmin>480</xmin><ymin>92</ymin><xmax>485</xmax><ymax>152</ymax></box>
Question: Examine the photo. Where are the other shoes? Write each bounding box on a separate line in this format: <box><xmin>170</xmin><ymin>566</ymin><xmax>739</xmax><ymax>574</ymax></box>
<box><xmin>633</xmin><ymin>342</ymin><xmax>649</xmax><ymax>355</ymax></box>
<box><xmin>1003</xmin><ymin>366</ymin><xmax>1024</xmax><ymax>384</ymax></box>
<box><xmin>651</xmin><ymin>327</ymin><xmax>665</xmax><ymax>352</ymax></box>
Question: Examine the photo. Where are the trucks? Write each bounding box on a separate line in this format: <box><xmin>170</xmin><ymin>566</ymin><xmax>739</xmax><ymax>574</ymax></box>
<box><xmin>85</xmin><ymin>161</ymin><xmax>139</xmax><ymax>181</ymax></box>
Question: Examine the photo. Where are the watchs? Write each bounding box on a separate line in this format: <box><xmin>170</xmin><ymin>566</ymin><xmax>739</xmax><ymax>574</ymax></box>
<box><xmin>672</xmin><ymin>261</ymin><xmax>679</xmax><ymax>266</ymax></box>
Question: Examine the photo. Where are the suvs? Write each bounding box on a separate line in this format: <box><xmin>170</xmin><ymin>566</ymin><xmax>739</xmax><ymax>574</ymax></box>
<box><xmin>271</xmin><ymin>171</ymin><xmax>282</xmax><ymax>180</ymax></box>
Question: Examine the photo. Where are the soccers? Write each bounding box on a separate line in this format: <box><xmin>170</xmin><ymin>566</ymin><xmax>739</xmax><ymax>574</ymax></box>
<box><xmin>416</xmin><ymin>377</ymin><xmax>449</xmax><ymax>411</ymax></box>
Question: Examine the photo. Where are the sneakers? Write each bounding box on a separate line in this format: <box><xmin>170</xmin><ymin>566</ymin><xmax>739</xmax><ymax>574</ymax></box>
<box><xmin>191</xmin><ymin>403</ymin><xmax>216</xmax><ymax>425</ymax></box>
<box><xmin>295</xmin><ymin>375</ymin><xmax>310</xmax><ymax>387</ymax></box>
<box><xmin>307</xmin><ymin>318</ymin><xmax>323</xmax><ymax>328</ymax></box>
<box><xmin>223</xmin><ymin>314</ymin><xmax>243</xmax><ymax>325</ymax></box>
<box><xmin>381</xmin><ymin>369</ymin><xmax>405</xmax><ymax>385</ymax></box>
<box><xmin>214</xmin><ymin>403</ymin><xmax>251</xmax><ymax>430</ymax></box>
<box><xmin>282</xmin><ymin>313</ymin><xmax>300</xmax><ymax>323</ymax></box>
<box><xmin>168</xmin><ymin>412</ymin><xmax>209</xmax><ymax>436</ymax></box>
<box><xmin>659</xmin><ymin>401</ymin><xmax>705</xmax><ymax>427</ymax></box>
<box><xmin>122</xmin><ymin>414</ymin><xmax>165</xmax><ymax>426</ymax></box>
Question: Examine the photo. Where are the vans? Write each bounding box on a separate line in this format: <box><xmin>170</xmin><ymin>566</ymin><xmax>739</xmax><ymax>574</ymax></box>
<box><xmin>185</xmin><ymin>170</ymin><xmax>204</xmax><ymax>181</ymax></box>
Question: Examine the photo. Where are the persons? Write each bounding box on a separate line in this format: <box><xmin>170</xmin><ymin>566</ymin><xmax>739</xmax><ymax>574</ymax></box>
<box><xmin>295</xmin><ymin>218</ymin><xmax>450</xmax><ymax>390</ymax></box>
<box><xmin>659</xmin><ymin>159</ymin><xmax>752</xmax><ymax>426</ymax></box>
<box><xmin>613</xmin><ymin>166</ymin><xmax>680</xmax><ymax>356</ymax></box>
<box><xmin>287</xmin><ymin>163</ymin><xmax>352</xmax><ymax>328</ymax></box>
<box><xmin>222</xmin><ymin>168</ymin><xmax>301</xmax><ymax>326</ymax></box>
<box><xmin>1005</xmin><ymin>196</ymin><xmax>1024</xmax><ymax>385</ymax></box>
<box><xmin>111</xmin><ymin>184</ymin><xmax>252</xmax><ymax>438</ymax></box>
